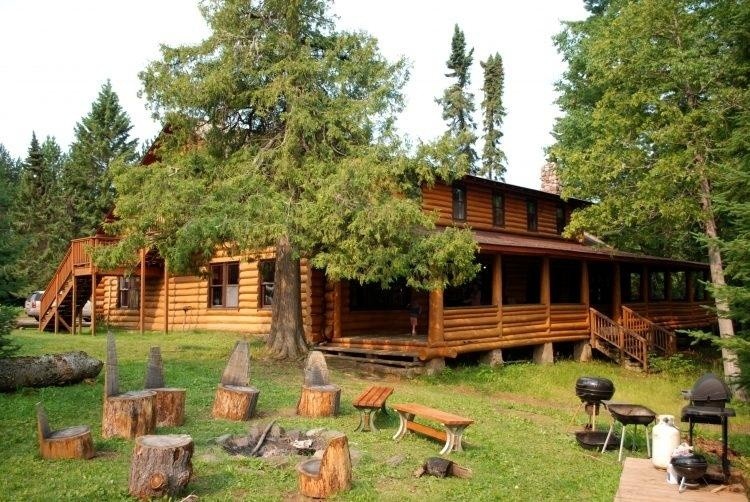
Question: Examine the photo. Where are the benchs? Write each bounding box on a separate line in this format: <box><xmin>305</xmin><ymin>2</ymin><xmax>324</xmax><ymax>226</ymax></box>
<box><xmin>391</xmin><ymin>402</ymin><xmax>475</xmax><ymax>455</ymax></box>
<box><xmin>352</xmin><ymin>384</ymin><xmax>396</xmax><ymax>436</ymax></box>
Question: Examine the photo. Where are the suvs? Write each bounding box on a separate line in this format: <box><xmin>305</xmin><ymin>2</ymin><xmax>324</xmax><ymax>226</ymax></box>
<box><xmin>23</xmin><ymin>288</ymin><xmax>92</xmax><ymax>322</ymax></box>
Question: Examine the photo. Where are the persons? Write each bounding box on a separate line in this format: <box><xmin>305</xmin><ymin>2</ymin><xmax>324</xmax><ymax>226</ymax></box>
<box><xmin>405</xmin><ymin>296</ymin><xmax>421</xmax><ymax>336</ymax></box>
<box><xmin>468</xmin><ymin>283</ymin><xmax>481</xmax><ymax>306</ymax></box>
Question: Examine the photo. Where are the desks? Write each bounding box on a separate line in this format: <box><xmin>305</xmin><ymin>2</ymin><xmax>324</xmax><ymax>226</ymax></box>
<box><xmin>609</xmin><ymin>455</ymin><xmax>750</xmax><ymax>502</ymax></box>
